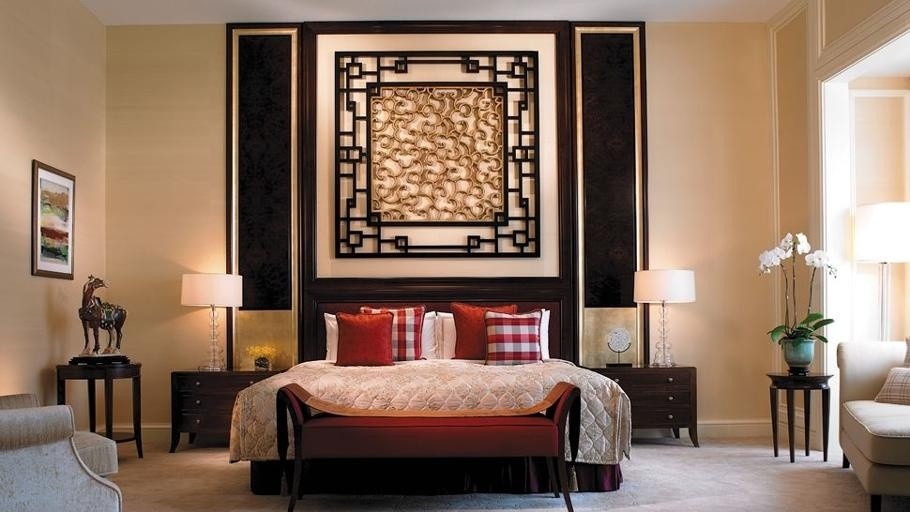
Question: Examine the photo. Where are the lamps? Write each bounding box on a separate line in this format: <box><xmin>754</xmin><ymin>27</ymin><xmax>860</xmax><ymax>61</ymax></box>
<box><xmin>634</xmin><ymin>269</ymin><xmax>696</xmax><ymax>369</ymax></box>
<box><xmin>180</xmin><ymin>273</ymin><xmax>244</xmax><ymax>371</ymax></box>
<box><xmin>853</xmin><ymin>204</ymin><xmax>909</xmax><ymax>339</ymax></box>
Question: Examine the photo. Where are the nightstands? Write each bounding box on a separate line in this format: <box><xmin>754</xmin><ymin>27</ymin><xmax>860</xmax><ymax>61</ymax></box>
<box><xmin>592</xmin><ymin>364</ymin><xmax>700</xmax><ymax>449</ymax></box>
<box><xmin>168</xmin><ymin>367</ymin><xmax>287</xmax><ymax>453</ymax></box>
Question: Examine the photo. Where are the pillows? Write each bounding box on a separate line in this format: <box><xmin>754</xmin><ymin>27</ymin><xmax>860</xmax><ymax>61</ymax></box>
<box><xmin>876</xmin><ymin>367</ymin><xmax>910</xmax><ymax>406</ymax></box>
<box><xmin>320</xmin><ymin>300</ymin><xmax>552</xmax><ymax>367</ymax></box>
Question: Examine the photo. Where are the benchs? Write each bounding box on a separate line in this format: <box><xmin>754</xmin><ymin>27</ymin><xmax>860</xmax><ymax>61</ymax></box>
<box><xmin>276</xmin><ymin>382</ymin><xmax>583</xmax><ymax>512</ymax></box>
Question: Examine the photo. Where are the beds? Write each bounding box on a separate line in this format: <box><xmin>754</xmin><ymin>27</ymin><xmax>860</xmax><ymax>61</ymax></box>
<box><xmin>229</xmin><ymin>280</ymin><xmax>634</xmax><ymax>497</ymax></box>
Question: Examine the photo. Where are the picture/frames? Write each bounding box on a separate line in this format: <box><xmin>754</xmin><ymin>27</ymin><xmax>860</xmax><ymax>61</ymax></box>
<box><xmin>29</xmin><ymin>158</ymin><xmax>78</xmax><ymax>280</ymax></box>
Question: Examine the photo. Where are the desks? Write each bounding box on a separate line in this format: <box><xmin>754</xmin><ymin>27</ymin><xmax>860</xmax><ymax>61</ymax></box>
<box><xmin>55</xmin><ymin>363</ymin><xmax>145</xmax><ymax>459</ymax></box>
<box><xmin>767</xmin><ymin>372</ymin><xmax>834</xmax><ymax>464</ymax></box>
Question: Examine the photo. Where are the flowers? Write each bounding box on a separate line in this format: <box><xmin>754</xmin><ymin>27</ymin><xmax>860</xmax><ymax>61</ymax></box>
<box><xmin>756</xmin><ymin>232</ymin><xmax>837</xmax><ymax>350</ymax></box>
<box><xmin>245</xmin><ymin>345</ymin><xmax>278</xmax><ymax>363</ymax></box>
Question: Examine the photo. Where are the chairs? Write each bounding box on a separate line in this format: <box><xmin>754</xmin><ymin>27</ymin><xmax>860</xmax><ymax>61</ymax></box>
<box><xmin>0</xmin><ymin>392</ymin><xmax>119</xmax><ymax>476</ymax></box>
<box><xmin>0</xmin><ymin>404</ymin><xmax>123</xmax><ymax>512</ymax></box>
<box><xmin>836</xmin><ymin>337</ymin><xmax>910</xmax><ymax>511</ymax></box>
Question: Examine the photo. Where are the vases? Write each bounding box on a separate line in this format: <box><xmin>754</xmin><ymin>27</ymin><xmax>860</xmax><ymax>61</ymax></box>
<box><xmin>781</xmin><ymin>338</ymin><xmax>819</xmax><ymax>369</ymax></box>
<box><xmin>254</xmin><ymin>357</ymin><xmax>272</xmax><ymax>371</ymax></box>
